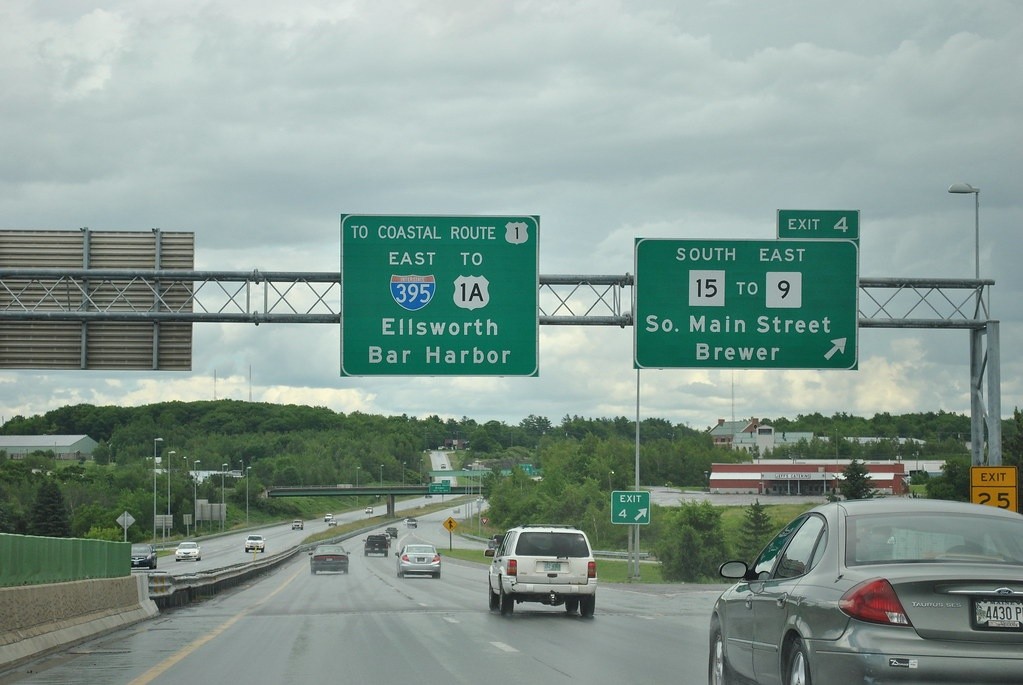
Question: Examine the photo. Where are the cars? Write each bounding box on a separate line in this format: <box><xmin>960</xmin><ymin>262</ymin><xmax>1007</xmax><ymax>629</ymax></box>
<box><xmin>323</xmin><ymin>513</ymin><xmax>334</xmax><ymax>522</ymax></box>
<box><xmin>245</xmin><ymin>535</ymin><xmax>266</xmax><ymax>553</ymax></box>
<box><xmin>292</xmin><ymin>520</ymin><xmax>303</xmax><ymax>530</ymax></box>
<box><xmin>441</xmin><ymin>464</ymin><xmax>446</xmax><ymax>468</ymax></box>
<box><xmin>708</xmin><ymin>498</ymin><xmax>1023</xmax><ymax>685</ymax></box>
<box><xmin>308</xmin><ymin>545</ymin><xmax>351</xmax><ymax>574</ymax></box>
<box><xmin>362</xmin><ymin>535</ymin><xmax>389</xmax><ymax>557</ymax></box>
<box><xmin>404</xmin><ymin>519</ymin><xmax>417</xmax><ymax>528</ymax></box>
<box><xmin>488</xmin><ymin>533</ymin><xmax>503</xmax><ymax>550</ymax></box>
<box><xmin>379</xmin><ymin>533</ymin><xmax>392</xmax><ymax>548</ymax></box>
<box><xmin>365</xmin><ymin>506</ymin><xmax>373</xmax><ymax>514</ymax></box>
<box><xmin>328</xmin><ymin>519</ymin><xmax>337</xmax><ymax>526</ymax></box>
<box><xmin>395</xmin><ymin>545</ymin><xmax>442</xmax><ymax>579</ymax></box>
<box><xmin>174</xmin><ymin>542</ymin><xmax>202</xmax><ymax>562</ymax></box>
<box><xmin>131</xmin><ymin>544</ymin><xmax>157</xmax><ymax>570</ymax></box>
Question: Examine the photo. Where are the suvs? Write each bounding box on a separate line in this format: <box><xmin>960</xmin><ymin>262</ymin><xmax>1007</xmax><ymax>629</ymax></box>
<box><xmin>485</xmin><ymin>523</ymin><xmax>598</xmax><ymax>617</ymax></box>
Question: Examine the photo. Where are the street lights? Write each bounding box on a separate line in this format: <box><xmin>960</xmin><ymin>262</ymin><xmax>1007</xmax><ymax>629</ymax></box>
<box><xmin>462</xmin><ymin>460</ymin><xmax>482</xmax><ymax>536</ymax></box>
<box><xmin>153</xmin><ymin>436</ymin><xmax>424</xmax><ymax>550</ymax></box>
<box><xmin>948</xmin><ymin>182</ymin><xmax>981</xmax><ymax>277</ymax></box>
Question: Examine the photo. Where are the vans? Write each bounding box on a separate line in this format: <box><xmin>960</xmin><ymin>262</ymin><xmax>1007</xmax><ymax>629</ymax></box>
<box><xmin>385</xmin><ymin>527</ymin><xmax>399</xmax><ymax>539</ymax></box>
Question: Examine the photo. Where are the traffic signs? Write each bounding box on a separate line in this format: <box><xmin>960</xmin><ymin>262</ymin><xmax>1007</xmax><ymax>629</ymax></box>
<box><xmin>633</xmin><ymin>238</ymin><xmax>857</xmax><ymax>370</ymax></box>
<box><xmin>610</xmin><ymin>491</ymin><xmax>650</xmax><ymax>525</ymax></box>
